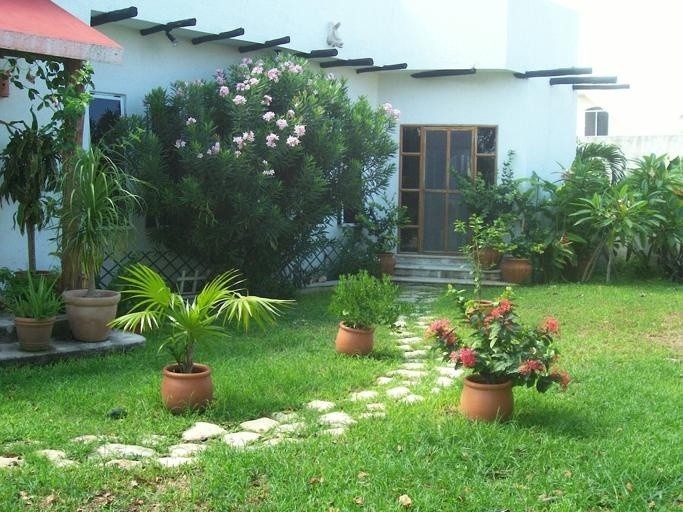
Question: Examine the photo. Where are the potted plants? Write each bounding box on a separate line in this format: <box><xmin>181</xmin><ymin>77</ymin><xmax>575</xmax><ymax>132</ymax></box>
<box><xmin>5</xmin><ymin>264</ymin><xmax>68</xmax><ymax>351</ymax></box>
<box><xmin>492</xmin><ymin>230</ymin><xmax>545</xmax><ymax>283</ymax></box>
<box><xmin>354</xmin><ymin>190</ymin><xmax>411</xmax><ymax>273</ymax></box>
<box><xmin>0</xmin><ymin>107</ymin><xmax>56</xmax><ymax>283</ymax></box>
<box><xmin>453</xmin><ymin>214</ymin><xmax>518</xmax><ymax>321</ymax></box>
<box><xmin>43</xmin><ymin>128</ymin><xmax>156</xmax><ymax>342</ymax></box>
<box><xmin>450</xmin><ymin>149</ymin><xmax>529</xmax><ymax>272</ymax></box>
<box><xmin>324</xmin><ymin>270</ymin><xmax>416</xmax><ymax>356</ymax></box>
<box><xmin>106</xmin><ymin>262</ymin><xmax>298</xmax><ymax>417</ymax></box>
<box><xmin>532</xmin><ymin>140</ymin><xmax>627</xmax><ymax>283</ymax></box>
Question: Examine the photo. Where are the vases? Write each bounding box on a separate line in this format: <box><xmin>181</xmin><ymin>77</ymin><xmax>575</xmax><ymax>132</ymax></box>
<box><xmin>459</xmin><ymin>375</ymin><xmax>514</xmax><ymax>422</ymax></box>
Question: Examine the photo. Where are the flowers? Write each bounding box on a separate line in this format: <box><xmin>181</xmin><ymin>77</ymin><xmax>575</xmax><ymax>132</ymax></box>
<box><xmin>425</xmin><ymin>282</ymin><xmax>570</xmax><ymax>394</ymax></box>
